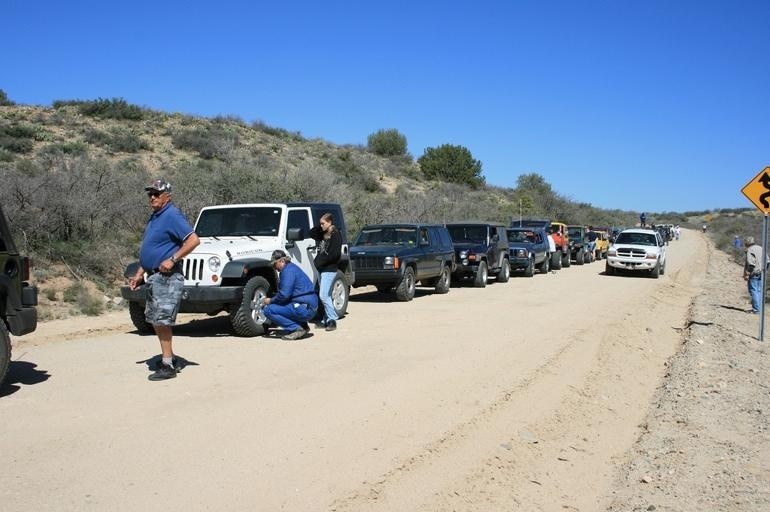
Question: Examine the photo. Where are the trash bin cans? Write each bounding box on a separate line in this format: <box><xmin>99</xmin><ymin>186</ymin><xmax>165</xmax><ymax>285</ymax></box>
<box><xmin>170</xmin><ymin>256</ymin><xmax>177</xmax><ymax>262</ymax></box>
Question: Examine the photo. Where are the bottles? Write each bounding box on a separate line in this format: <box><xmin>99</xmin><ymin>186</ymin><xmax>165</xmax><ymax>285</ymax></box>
<box><xmin>270</xmin><ymin>250</ymin><xmax>286</xmax><ymax>266</ymax></box>
<box><xmin>145</xmin><ymin>177</ymin><xmax>171</xmax><ymax>192</ymax></box>
<box><xmin>744</xmin><ymin>236</ymin><xmax>754</xmax><ymax>244</ymax></box>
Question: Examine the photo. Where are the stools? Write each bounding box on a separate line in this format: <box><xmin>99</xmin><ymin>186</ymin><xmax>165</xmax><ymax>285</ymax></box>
<box><xmin>147</xmin><ymin>268</ymin><xmax>159</xmax><ymax>276</ymax></box>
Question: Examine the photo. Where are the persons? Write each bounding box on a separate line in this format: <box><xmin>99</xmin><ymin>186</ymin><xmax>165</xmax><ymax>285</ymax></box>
<box><xmin>734</xmin><ymin>236</ymin><xmax>741</xmax><ymax>259</ymax></box>
<box><xmin>309</xmin><ymin>213</ymin><xmax>341</xmax><ymax>331</ymax></box>
<box><xmin>586</xmin><ymin>226</ymin><xmax>597</xmax><ymax>251</ymax></box>
<box><xmin>129</xmin><ymin>180</ymin><xmax>201</xmax><ymax>380</ymax></box>
<box><xmin>702</xmin><ymin>224</ymin><xmax>707</xmax><ymax>233</ymax></box>
<box><xmin>743</xmin><ymin>236</ymin><xmax>770</xmax><ymax>314</ymax></box>
<box><xmin>261</xmin><ymin>250</ymin><xmax>318</xmax><ymax>341</ymax></box>
<box><xmin>675</xmin><ymin>224</ymin><xmax>681</xmax><ymax>240</ymax></box>
<box><xmin>640</xmin><ymin>212</ymin><xmax>647</xmax><ymax>228</ymax></box>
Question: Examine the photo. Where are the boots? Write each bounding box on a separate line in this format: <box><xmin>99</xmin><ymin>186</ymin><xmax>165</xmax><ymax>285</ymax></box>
<box><xmin>120</xmin><ymin>199</ymin><xmax>358</xmax><ymax>336</ymax></box>
<box><xmin>0</xmin><ymin>208</ymin><xmax>41</xmax><ymax>388</ymax></box>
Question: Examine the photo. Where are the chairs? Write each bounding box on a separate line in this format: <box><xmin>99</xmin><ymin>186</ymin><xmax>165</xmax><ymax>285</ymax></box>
<box><xmin>282</xmin><ymin>319</ymin><xmax>336</xmax><ymax>340</ymax></box>
<box><xmin>148</xmin><ymin>357</ymin><xmax>177</xmax><ymax>381</ymax></box>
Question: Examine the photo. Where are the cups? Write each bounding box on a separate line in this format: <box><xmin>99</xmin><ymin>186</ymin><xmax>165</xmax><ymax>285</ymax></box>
<box><xmin>147</xmin><ymin>191</ymin><xmax>165</xmax><ymax>198</ymax></box>
